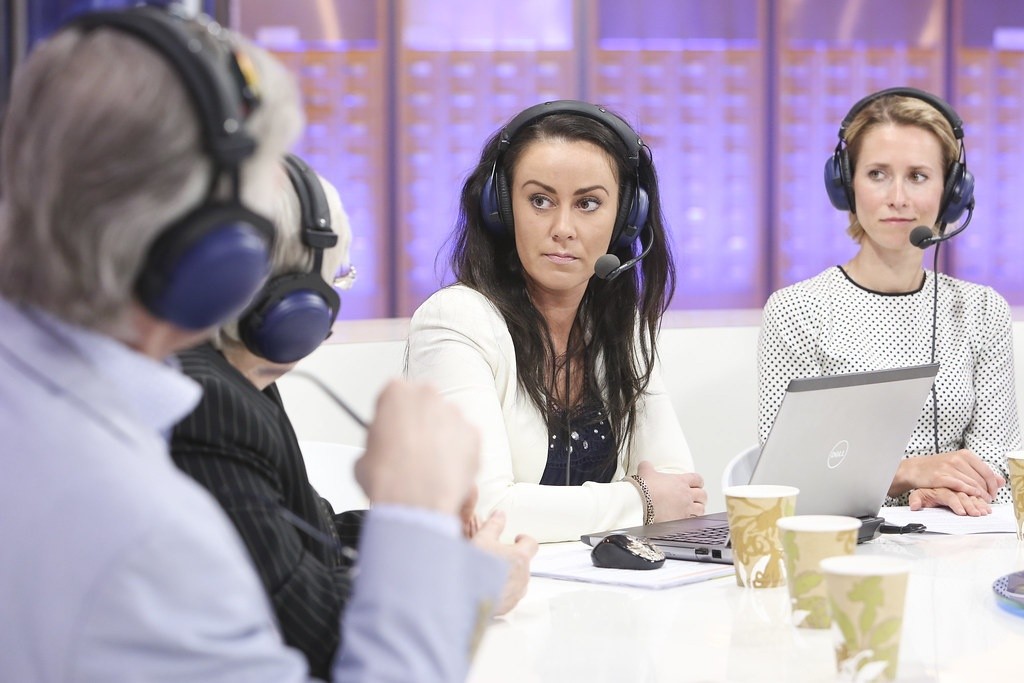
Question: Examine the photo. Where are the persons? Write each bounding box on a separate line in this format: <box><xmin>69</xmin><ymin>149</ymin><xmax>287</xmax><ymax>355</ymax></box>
<box><xmin>167</xmin><ymin>173</ymin><xmax>538</xmax><ymax>681</ymax></box>
<box><xmin>758</xmin><ymin>87</ymin><xmax>1023</xmax><ymax>517</ymax></box>
<box><xmin>405</xmin><ymin>98</ymin><xmax>709</xmax><ymax>545</ymax></box>
<box><xmin>0</xmin><ymin>5</ymin><xmax>508</xmax><ymax>683</ymax></box>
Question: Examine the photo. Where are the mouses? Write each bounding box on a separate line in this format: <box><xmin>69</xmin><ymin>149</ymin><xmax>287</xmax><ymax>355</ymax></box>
<box><xmin>591</xmin><ymin>533</ymin><xmax>668</xmax><ymax>570</ymax></box>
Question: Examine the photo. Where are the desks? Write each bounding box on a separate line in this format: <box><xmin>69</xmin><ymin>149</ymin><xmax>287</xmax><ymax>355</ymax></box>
<box><xmin>457</xmin><ymin>503</ymin><xmax>1024</xmax><ymax>682</ymax></box>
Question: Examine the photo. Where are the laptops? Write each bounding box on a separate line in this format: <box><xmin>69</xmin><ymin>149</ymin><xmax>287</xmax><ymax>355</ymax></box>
<box><xmin>580</xmin><ymin>361</ymin><xmax>942</xmax><ymax>564</ymax></box>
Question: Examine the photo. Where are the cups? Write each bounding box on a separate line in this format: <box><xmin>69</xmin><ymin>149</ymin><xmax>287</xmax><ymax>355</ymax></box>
<box><xmin>1006</xmin><ymin>451</ymin><xmax>1024</xmax><ymax>541</ymax></box>
<box><xmin>724</xmin><ymin>485</ymin><xmax>800</xmax><ymax>588</ymax></box>
<box><xmin>776</xmin><ymin>514</ymin><xmax>863</xmax><ymax>629</ymax></box>
<box><xmin>820</xmin><ymin>554</ymin><xmax>912</xmax><ymax>682</ymax></box>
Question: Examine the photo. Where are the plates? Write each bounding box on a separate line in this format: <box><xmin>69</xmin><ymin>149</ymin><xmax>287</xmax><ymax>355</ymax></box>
<box><xmin>993</xmin><ymin>570</ymin><xmax>1024</xmax><ymax>604</ymax></box>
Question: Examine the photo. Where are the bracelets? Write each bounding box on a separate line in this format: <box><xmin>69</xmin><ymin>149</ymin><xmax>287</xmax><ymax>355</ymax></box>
<box><xmin>632</xmin><ymin>475</ymin><xmax>654</xmax><ymax>525</ymax></box>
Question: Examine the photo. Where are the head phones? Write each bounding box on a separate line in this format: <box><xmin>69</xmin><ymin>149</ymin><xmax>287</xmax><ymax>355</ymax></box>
<box><xmin>238</xmin><ymin>155</ymin><xmax>341</xmax><ymax>364</ymax></box>
<box><xmin>479</xmin><ymin>98</ymin><xmax>649</xmax><ymax>252</ymax></box>
<box><xmin>824</xmin><ymin>86</ymin><xmax>975</xmax><ymax>226</ymax></box>
<box><xmin>65</xmin><ymin>6</ymin><xmax>276</xmax><ymax>331</ymax></box>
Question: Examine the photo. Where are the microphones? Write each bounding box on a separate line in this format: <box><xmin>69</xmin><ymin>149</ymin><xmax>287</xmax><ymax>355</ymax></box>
<box><xmin>594</xmin><ymin>221</ymin><xmax>653</xmax><ymax>282</ymax></box>
<box><xmin>909</xmin><ymin>198</ymin><xmax>975</xmax><ymax>249</ymax></box>
